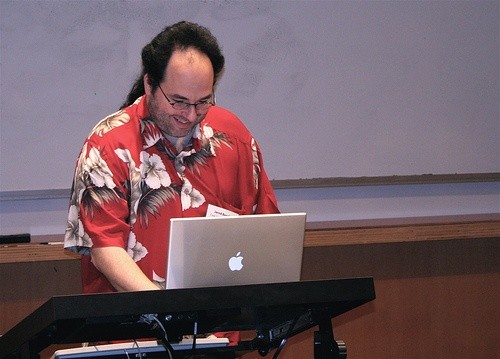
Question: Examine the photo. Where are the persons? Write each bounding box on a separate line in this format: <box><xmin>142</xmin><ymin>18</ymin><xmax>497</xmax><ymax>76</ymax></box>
<box><xmin>63</xmin><ymin>20</ymin><xmax>283</xmax><ymax>359</ymax></box>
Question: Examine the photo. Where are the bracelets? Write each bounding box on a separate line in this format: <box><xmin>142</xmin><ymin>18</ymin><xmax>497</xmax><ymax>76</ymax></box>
<box><xmin>152</xmin><ymin>281</ymin><xmax>166</xmax><ymax>292</ymax></box>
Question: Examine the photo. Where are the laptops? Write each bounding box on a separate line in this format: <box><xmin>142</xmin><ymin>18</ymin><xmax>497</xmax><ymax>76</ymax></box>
<box><xmin>166</xmin><ymin>212</ymin><xmax>307</xmax><ymax>289</ymax></box>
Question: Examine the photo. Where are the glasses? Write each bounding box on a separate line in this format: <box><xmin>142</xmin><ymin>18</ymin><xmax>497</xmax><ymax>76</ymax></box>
<box><xmin>158</xmin><ymin>84</ymin><xmax>216</xmax><ymax>111</ymax></box>
